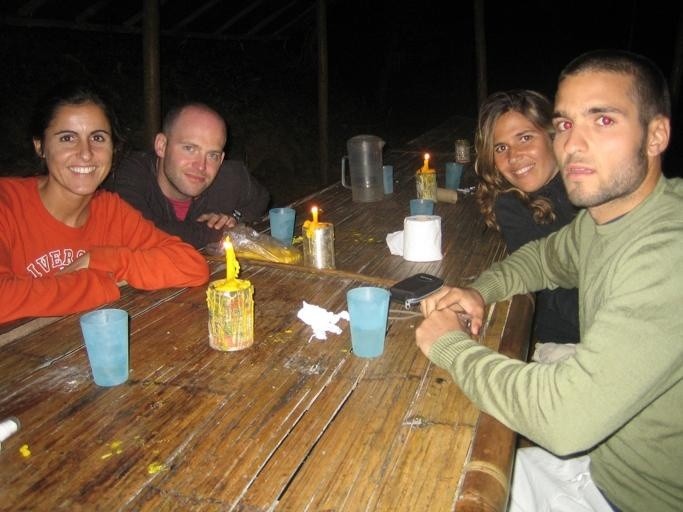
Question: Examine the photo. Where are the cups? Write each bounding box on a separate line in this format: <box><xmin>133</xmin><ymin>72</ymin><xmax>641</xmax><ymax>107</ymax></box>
<box><xmin>383</xmin><ymin>165</ymin><xmax>394</xmax><ymax>195</ymax></box>
<box><xmin>410</xmin><ymin>200</ymin><xmax>433</xmax><ymax>215</ymax></box>
<box><xmin>79</xmin><ymin>308</ymin><xmax>129</xmax><ymax>387</ymax></box>
<box><xmin>269</xmin><ymin>208</ymin><xmax>296</xmax><ymax>248</ymax></box>
<box><xmin>346</xmin><ymin>287</ymin><xmax>390</xmax><ymax>359</ymax></box>
<box><xmin>446</xmin><ymin>162</ymin><xmax>463</xmax><ymax>189</ymax></box>
<box><xmin>455</xmin><ymin>138</ymin><xmax>471</xmax><ymax>163</ymax></box>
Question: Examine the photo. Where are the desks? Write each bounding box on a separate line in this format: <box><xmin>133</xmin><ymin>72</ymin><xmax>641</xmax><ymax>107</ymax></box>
<box><xmin>0</xmin><ymin>115</ymin><xmax>536</xmax><ymax>511</ymax></box>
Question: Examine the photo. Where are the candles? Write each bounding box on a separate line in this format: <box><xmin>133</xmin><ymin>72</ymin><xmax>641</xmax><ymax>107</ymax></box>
<box><xmin>414</xmin><ymin>153</ymin><xmax>438</xmax><ymax>203</ymax></box>
<box><xmin>302</xmin><ymin>206</ymin><xmax>336</xmax><ymax>268</ymax></box>
<box><xmin>207</xmin><ymin>236</ymin><xmax>256</xmax><ymax>352</ymax></box>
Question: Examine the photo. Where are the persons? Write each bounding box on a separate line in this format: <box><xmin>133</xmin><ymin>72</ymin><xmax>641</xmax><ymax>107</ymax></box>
<box><xmin>415</xmin><ymin>49</ymin><xmax>682</xmax><ymax>512</ymax></box>
<box><xmin>100</xmin><ymin>99</ymin><xmax>272</xmax><ymax>250</ymax></box>
<box><xmin>435</xmin><ymin>180</ymin><xmax>477</xmax><ymax>204</ymax></box>
<box><xmin>0</xmin><ymin>82</ymin><xmax>210</xmax><ymax>326</ymax></box>
<box><xmin>473</xmin><ymin>90</ymin><xmax>584</xmax><ymax>342</ymax></box>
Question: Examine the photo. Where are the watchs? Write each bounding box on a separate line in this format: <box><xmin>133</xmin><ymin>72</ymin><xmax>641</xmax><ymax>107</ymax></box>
<box><xmin>228</xmin><ymin>208</ymin><xmax>242</xmax><ymax>224</ymax></box>
